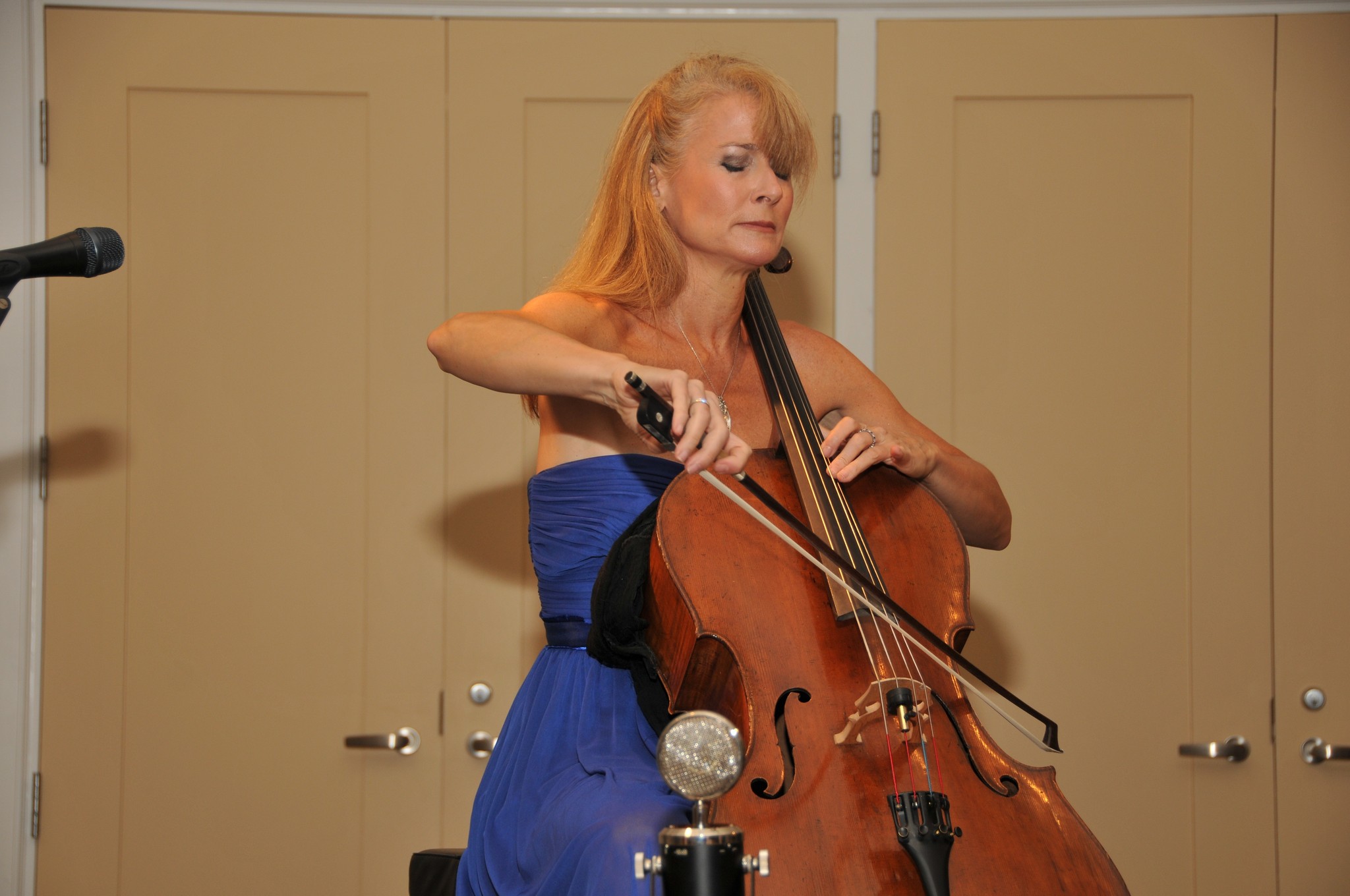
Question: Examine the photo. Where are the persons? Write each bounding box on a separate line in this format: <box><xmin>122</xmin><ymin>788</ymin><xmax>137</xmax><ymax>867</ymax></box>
<box><xmin>428</xmin><ymin>54</ymin><xmax>1013</xmax><ymax>896</ymax></box>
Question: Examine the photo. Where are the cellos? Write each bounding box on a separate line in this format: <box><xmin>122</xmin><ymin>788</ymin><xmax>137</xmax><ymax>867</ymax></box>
<box><xmin>646</xmin><ymin>242</ymin><xmax>1133</xmax><ymax>896</ymax></box>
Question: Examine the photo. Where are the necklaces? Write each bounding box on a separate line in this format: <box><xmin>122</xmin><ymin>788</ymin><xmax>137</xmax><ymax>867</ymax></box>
<box><xmin>664</xmin><ymin>299</ymin><xmax>741</xmax><ymax>431</ymax></box>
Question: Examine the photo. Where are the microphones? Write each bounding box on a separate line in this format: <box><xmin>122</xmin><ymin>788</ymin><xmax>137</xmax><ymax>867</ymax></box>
<box><xmin>0</xmin><ymin>227</ymin><xmax>125</xmax><ymax>286</ymax></box>
<box><xmin>656</xmin><ymin>711</ymin><xmax>746</xmax><ymax>799</ymax></box>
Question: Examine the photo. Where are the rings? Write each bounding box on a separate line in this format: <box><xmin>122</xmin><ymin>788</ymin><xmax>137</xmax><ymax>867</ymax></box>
<box><xmin>689</xmin><ymin>398</ymin><xmax>711</xmax><ymax>408</ymax></box>
<box><xmin>858</xmin><ymin>429</ymin><xmax>876</xmax><ymax>449</ymax></box>
